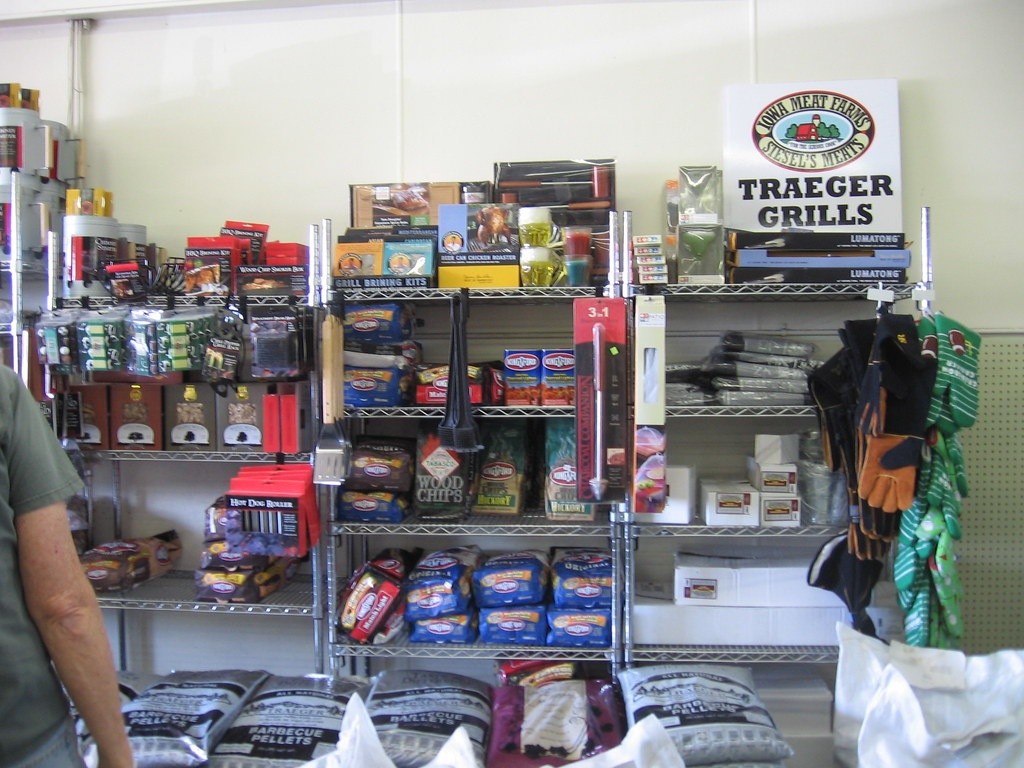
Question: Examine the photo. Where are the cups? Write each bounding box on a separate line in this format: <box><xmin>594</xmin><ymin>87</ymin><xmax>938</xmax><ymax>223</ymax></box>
<box><xmin>517</xmin><ymin>208</ymin><xmax>595</xmax><ymax>288</ymax></box>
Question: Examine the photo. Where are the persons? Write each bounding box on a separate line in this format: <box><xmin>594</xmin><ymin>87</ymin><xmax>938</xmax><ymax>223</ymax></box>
<box><xmin>0</xmin><ymin>363</ymin><xmax>138</xmax><ymax>768</ymax></box>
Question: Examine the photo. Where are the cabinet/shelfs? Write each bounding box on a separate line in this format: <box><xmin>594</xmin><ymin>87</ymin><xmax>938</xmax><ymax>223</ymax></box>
<box><xmin>322</xmin><ymin>216</ymin><xmax>621</xmax><ymax>705</ymax></box>
<box><xmin>623</xmin><ymin>211</ymin><xmax>935</xmax><ymax>692</ymax></box>
<box><xmin>47</xmin><ymin>230</ymin><xmax>322</xmax><ymax>686</ymax></box>
<box><xmin>0</xmin><ymin>171</ymin><xmax>66</xmax><ymax>392</ymax></box>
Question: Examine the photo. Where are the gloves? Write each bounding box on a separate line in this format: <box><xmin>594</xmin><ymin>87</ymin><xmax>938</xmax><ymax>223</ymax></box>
<box><xmin>807</xmin><ymin>310</ymin><xmax>982</xmax><ymax>652</ymax></box>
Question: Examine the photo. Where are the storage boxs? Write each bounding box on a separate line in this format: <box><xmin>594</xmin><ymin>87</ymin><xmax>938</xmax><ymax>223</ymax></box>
<box><xmin>638</xmin><ymin>461</ymin><xmax>805</xmax><ymax>607</ymax></box>
<box><xmin>753</xmin><ymin>666</ymin><xmax>833</xmax><ymax>768</ymax></box>
<box><xmin>63</xmin><ymin>377</ymin><xmax>320</xmax><ymax>559</ymax></box>
